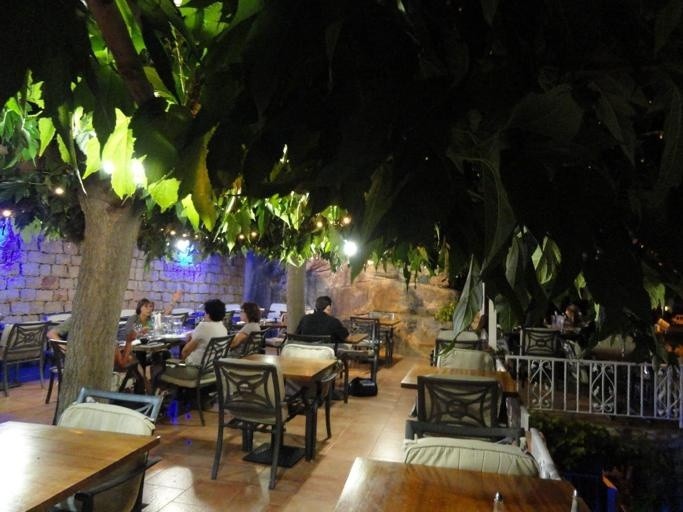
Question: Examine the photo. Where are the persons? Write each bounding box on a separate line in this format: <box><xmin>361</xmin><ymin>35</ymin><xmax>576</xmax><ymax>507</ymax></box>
<box><xmin>46</xmin><ymin>317</ymin><xmax>70</xmax><ymax>356</ymax></box>
<box><xmin>111</xmin><ymin>331</ymin><xmax>153</xmax><ymax>394</ymax></box>
<box><xmin>125</xmin><ymin>289</ymin><xmax>181</xmax><ymax>329</ymax></box>
<box><xmin>293</xmin><ymin>296</ymin><xmax>350</xmax><ymax>344</ymax></box>
<box><xmin>154</xmin><ymin>300</ymin><xmax>228</xmax><ymax>415</ymax></box>
<box><xmin>229</xmin><ymin>303</ymin><xmax>262</xmax><ymax>356</ymax></box>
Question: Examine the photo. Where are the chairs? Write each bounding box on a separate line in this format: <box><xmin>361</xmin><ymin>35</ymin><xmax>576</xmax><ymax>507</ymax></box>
<box><xmin>240</xmin><ymin>326</ymin><xmax>272</xmax><ymax>357</ymax></box>
<box><xmin>44</xmin><ymin>312</ymin><xmax>73</xmax><ymax>332</ymax></box>
<box><xmin>110</xmin><ymin>358</ymin><xmax>146</xmax><ymax>413</ymax></box>
<box><xmin>1</xmin><ymin>320</ymin><xmax>49</xmax><ymax>397</ymax></box>
<box><xmin>282</xmin><ymin>334</ymin><xmax>350</xmax><ymax>403</ymax></box>
<box><xmin>405</xmin><ymin>373</ymin><xmax>526</xmax><ymax>449</ymax></box>
<box><xmin>45</xmin><ymin>337</ymin><xmax>67</xmax><ymax>403</ymax></box>
<box><xmin>280</xmin><ymin>340</ymin><xmax>335</xmax><ymax>439</ymax></box>
<box><xmin>367</xmin><ymin>310</ymin><xmax>397</xmax><ymax>365</ymax></box>
<box><xmin>349</xmin><ymin>316</ymin><xmax>380</xmax><ymax>382</ymax></box>
<box><xmin>211</xmin><ymin>357</ymin><xmax>315</xmax><ymax>489</ymax></box>
<box><xmin>50</xmin><ymin>387</ymin><xmax>165</xmax><ymax>511</ymax></box>
<box><xmin>399</xmin><ymin>421</ymin><xmax>542</xmax><ymax>478</ymax></box>
<box><xmin>436</xmin><ymin>347</ymin><xmax>497</xmax><ymax>374</ymax></box>
<box><xmin>265</xmin><ymin>313</ymin><xmax>287</xmax><ymax>355</ymax></box>
<box><xmin>156</xmin><ymin>335</ymin><xmax>236</xmax><ymax>427</ymax></box>
<box><xmin>517</xmin><ymin>326</ymin><xmax>563</xmax><ymax>389</ymax></box>
<box><xmin>222</xmin><ymin>310</ymin><xmax>236</xmax><ymax>334</ymax></box>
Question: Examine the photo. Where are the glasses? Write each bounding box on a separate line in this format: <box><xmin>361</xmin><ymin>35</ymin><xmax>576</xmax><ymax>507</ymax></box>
<box><xmin>238</xmin><ymin>309</ymin><xmax>248</xmax><ymax>313</ymax></box>
